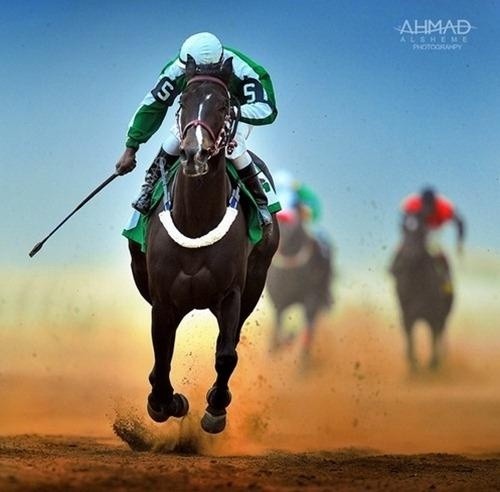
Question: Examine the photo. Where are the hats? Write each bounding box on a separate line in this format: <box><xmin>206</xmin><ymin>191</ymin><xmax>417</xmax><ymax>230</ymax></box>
<box><xmin>175</xmin><ymin>31</ymin><xmax>224</xmax><ymax>69</ymax></box>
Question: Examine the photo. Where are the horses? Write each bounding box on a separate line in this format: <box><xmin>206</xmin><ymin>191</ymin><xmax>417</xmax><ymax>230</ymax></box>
<box><xmin>395</xmin><ymin>217</ymin><xmax>452</xmax><ymax>376</ymax></box>
<box><xmin>130</xmin><ymin>53</ymin><xmax>279</xmax><ymax>434</ymax></box>
<box><xmin>266</xmin><ymin>208</ymin><xmax>329</xmax><ymax>363</ymax></box>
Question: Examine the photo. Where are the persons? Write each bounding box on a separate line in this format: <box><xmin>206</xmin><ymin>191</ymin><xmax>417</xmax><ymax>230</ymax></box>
<box><xmin>267</xmin><ymin>163</ymin><xmax>338</xmax><ymax>309</ymax></box>
<box><xmin>115</xmin><ymin>33</ymin><xmax>279</xmax><ymax>263</ymax></box>
<box><xmin>400</xmin><ymin>185</ymin><xmax>466</xmax><ymax>306</ymax></box>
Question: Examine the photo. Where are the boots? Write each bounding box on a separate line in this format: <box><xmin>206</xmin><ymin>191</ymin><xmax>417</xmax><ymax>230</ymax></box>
<box><xmin>236</xmin><ymin>162</ymin><xmax>273</xmax><ymax>227</ymax></box>
<box><xmin>133</xmin><ymin>144</ymin><xmax>181</xmax><ymax>214</ymax></box>
